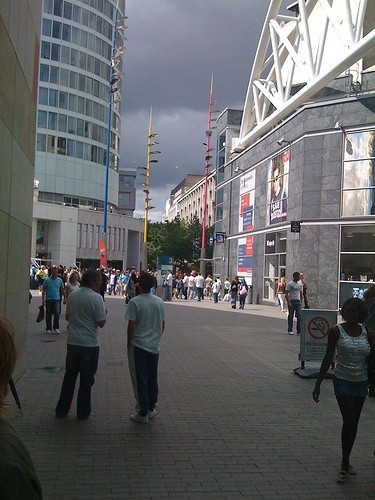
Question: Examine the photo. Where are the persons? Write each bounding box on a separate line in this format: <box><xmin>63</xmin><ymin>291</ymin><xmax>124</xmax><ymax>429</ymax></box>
<box><xmin>1</xmin><ymin>318</ymin><xmax>42</xmax><ymax>499</ymax></box>
<box><xmin>296</xmin><ymin>273</ymin><xmax>310</xmax><ymax>309</ymax></box>
<box><xmin>27</xmin><ymin>260</ymin><xmax>251</xmax><ymax>309</ymax></box>
<box><xmin>270</xmin><ymin>161</ymin><xmax>288</xmax><ymax>224</ymax></box>
<box><xmin>41</xmin><ymin>266</ymin><xmax>67</xmax><ymax>335</ymax></box>
<box><xmin>55</xmin><ymin>269</ymin><xmax>109</xmax><ymax>420</ymax></box>
<box><xmin>361</xmin><ymin>286</ymin><xmax>375</xmax><ymax>397</ymax></box>
<box><xmin>124</xmin><ymin>272</ymin><xmax>167</xmax><ymax>423</ymax></box>
<box><xmin>62</xmin><ymin>272</ymin><xmax>83</xmax><ymax>330</ymax></box>
<box><xmin>285</xmin><ymin>272</ymin><xmax>304</xmax><ymax>335</ymax></box>
<box><xmin>312</xmin><ymin>297</ymin><xmax>375</xmax><ymax>483</ymax></box>
<box><xmin>276</xmin><ymin>276</ymin><xmax>287</xmax><ymax>312</ymax></box>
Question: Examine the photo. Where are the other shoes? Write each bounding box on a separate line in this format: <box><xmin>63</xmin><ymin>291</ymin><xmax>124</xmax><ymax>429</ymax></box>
<box><xmin>163</xmin><ymin>295</ymin><xmax>289</xmax><ymax>312</ymax></box>
<box><xmin>56</xmin><ymin>413</ymin><xmax>89</xmax><ymax>420</ymax></box>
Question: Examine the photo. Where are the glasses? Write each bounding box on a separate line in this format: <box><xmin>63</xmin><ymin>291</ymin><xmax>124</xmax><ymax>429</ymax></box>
<box><xmin>273</xmin><ymin>175</ymin><xmax>279</xmax><ymax>183</ymax></box>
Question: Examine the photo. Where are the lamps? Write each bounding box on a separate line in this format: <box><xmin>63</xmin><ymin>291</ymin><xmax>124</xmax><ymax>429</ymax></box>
<box><xmin>277</xmin><ymin>138</ymin><xmax>290</xmax><ymax>146</ymax></box>
<box><xmin>335</xmin><ymin>121</ymin><xmax>342</xmax><ymax>130</ymax></box>
<box><xmin>235</xmin><ymin>167</ymin><xmax>244</xmax><ymax>172</ymax></box>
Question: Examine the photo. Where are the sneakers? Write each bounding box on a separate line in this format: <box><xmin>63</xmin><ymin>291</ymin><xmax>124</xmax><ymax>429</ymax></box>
<box><xmin>46</xmin><ymin>328</ymin><xmax>60</xmax><ymax>333</ymax></box>
<box><xmin>336</xmin><ymin>461</ymin><xmax>356</xmax><ymax>482</ymax></box>
<box><xmin>288</xmin><ymin>331</ymin><xmax>294</xmax><ymax>335</ymax></box>
<box><xmin>129</xmin><ymin>407</ymin><xmax>157</xmax><ymax>423</ymax></box>
